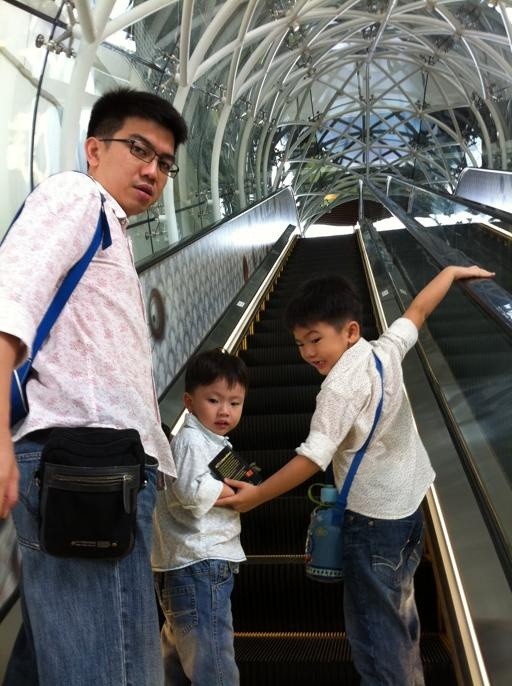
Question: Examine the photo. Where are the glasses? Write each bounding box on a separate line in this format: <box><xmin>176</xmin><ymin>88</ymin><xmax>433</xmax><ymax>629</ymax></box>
<box><xmin>100</xmin><ymin>137</ymin><xmax>180</xmax><ymax>179</ymax></box>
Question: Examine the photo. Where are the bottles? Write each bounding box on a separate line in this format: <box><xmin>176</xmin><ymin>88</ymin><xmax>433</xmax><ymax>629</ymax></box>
<box><xmin>303</xmin><ymin>483</ymin><xmax>344</xmax><ymax>584</ymax></box>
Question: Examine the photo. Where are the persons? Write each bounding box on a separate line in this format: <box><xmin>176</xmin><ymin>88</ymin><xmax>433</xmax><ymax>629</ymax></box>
<box><xmin>148</xmin><ymin>347</ymin><xmax>247</xmax><ymax>685</ymax></box>
<box><xmin>214</xmin><ymin>265</ymin><xmax>496</xmax><ymax>685</ymax></box>
<box><xmin>0</xmin><ymin>87</ymin><xmax>187</xmax><ymax>686</ymax></box>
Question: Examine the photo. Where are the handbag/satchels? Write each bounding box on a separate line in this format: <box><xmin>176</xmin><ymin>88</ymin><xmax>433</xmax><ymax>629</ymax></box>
<box><xmin>0</xmin><ymin>169</ymin><xmax>113</xmax><ymax>428</ymax></box>
<box><xmin>32</xmin><ymin>424</ymin><xmax>149</xmax><ymax>562</ymax></box>
